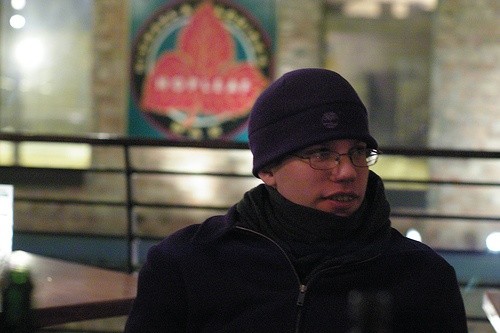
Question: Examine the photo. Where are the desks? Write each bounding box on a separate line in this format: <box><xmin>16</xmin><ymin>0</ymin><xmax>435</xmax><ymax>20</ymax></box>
<box><xmin>0</xmin><ymin>250</ymin><xmax>138</xmax><ymax>327</ymax></box>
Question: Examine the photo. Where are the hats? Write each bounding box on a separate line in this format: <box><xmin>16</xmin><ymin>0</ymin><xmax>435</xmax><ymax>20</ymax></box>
<box><xmin>248</xmin><ymin>68</ymin><xmax>379</xmax><ymax>179</ymax></box>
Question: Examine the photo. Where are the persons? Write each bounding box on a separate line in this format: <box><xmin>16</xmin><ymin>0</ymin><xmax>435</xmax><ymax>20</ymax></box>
<box><xmin>124</xmin><ymin>66</ymin><xmax>469</xmax><ymax>332</ymax></box>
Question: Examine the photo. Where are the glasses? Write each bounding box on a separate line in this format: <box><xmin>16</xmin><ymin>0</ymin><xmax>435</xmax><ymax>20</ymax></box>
<box><xmin>285</xmin><ymin>147</ymin><xmax>382</xmax><ymax>171</ymax></box>
